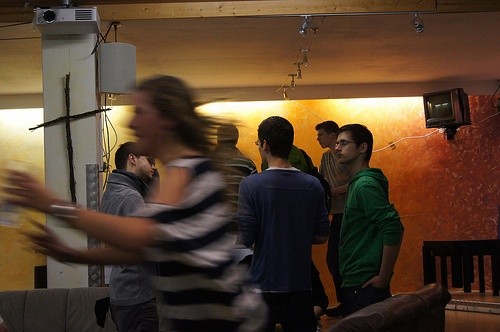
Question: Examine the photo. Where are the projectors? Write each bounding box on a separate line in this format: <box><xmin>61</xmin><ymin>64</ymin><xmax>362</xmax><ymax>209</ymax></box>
<box><xmin>36</xmin><ymin>7</ymin><xmax>101</xmax><ymax>36</ymax></box>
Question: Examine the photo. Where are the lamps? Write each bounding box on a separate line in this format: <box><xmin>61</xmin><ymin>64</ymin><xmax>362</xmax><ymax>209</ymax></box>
<box><xmin>282</xmin><ymin>15</ymin><xmax>319</xmax><ymax>100</ymax></box>
<box><xmin>97</xmin><ymin>22</ymin><xmax>137</xmax><ymax>94</ymax></box>
<box><xmin>410</xmin><ymin>14</ymin><xmax>425</xmax><ymax>33</ymax></box>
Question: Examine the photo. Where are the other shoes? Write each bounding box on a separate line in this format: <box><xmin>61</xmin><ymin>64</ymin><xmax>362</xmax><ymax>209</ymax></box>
<box><xmin>325</xmin><ymin>303</ymin><xmax>342</xmax><ymax>317</ymax></box>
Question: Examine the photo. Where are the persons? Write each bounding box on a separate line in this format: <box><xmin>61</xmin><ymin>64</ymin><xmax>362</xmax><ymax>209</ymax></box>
<box><xmin>0</xmin><ymin>73</ymin><xmax>269</xmax><ymax>332</ymax></box>
<box><xmin>261</xmin><ymin>146</ymin><xmax>329</xmax><ymax>317</ymax></box>
<box><xmin>101</xmin><ymin>142</ymin><xmax>159</xmax><ymax>332</ymax></box>
<box><xmin>235</xmin><ymin>117</ymin><xmax>328</xmax><ymax>332</ymax></box>
<box><xmin>315</xmin><ymin>121</ymin><xmax>353</xmax><ymax>317</ymax></box>
<box><xmin>335</xmin><ymin>123</ymin><xmax>404</xmax><ymax>311</ymax></box>
<box><xmin>212</xmin><ymin>123</ymin><xmax>257</xmax><ymax>227</ymax></box>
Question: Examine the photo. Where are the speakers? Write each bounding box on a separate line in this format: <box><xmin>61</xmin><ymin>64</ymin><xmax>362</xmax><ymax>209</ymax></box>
<box><xmin>98</xmin><ymin>42</ymin><xmax>136</xmax><ymax>95</ymax></box>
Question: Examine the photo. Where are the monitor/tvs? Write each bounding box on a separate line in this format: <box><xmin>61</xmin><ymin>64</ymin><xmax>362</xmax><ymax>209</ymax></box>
<box><xmin>422</xmin><ymin>87</ymin><xmax>472</xmax><ymax>129</ymax></box>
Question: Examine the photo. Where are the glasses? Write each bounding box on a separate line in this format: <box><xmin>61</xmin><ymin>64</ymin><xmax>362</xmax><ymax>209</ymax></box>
<box><xmin>335</xmin><ymin>141</ymin><xmax>355</xmax><ymax>146</ymax></box>
<box><xmin>255</xmin><ymin>139</ymin><xmax>260</xmax><ymax>145</ymax></box>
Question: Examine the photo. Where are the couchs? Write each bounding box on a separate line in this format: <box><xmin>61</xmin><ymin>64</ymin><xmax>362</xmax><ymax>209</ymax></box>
<box><xmin>0</xmin><ymin>286</ymin><xmax>116</xmax><ymax>332</ymax></box>
<box><xmin>327</xmin><ymin>282</ymin><xmax>452</xmax><ymax>332</ymax></box>
